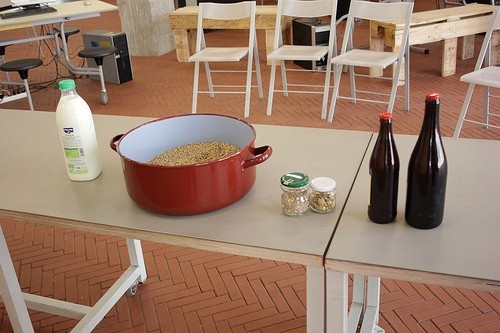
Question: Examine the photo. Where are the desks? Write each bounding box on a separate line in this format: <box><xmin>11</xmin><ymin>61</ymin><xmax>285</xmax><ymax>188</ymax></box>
<box><xmin>0</xmin><ymin>108</ymin><xmax>373</xmax><ymax>333</ymax></box>
<box><xmin>0</xmin><ymin>0</ymin><xmax>119</xmax><ymax>106</ymax></box>
<box><xmin>324</xmin><ymin>132</ymin><xmax>500</xmax><ymax>333</ymax></box>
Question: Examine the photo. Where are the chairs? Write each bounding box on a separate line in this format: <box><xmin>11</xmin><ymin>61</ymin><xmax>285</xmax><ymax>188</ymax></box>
<box><xmin>454</xmin><ymin>9</ymin><xmax>500</xmax><ymax>138</ymax></box>
<box><xmin>326</xmin><ymin>0</ymin><xmax>416</xmax><ymax>123</ymax></box>
<box><xmin>266</xmin><ymin>0</ymin><xmax>338</xmax><ymax>119</ymax></box>
<box><xmin>188</xmin><ymin>1</ymin><xmax>263</xmax><ymax>118</ymax></box>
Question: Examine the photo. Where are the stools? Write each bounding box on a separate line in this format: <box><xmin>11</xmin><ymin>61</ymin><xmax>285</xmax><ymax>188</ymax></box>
<box><xmin>0</xmin><ymin>57</ymin><xmax>44</xmax><ymax>111</ymax></box>
<box><xmin>0</xmin><ymin>38</ymin><xmax>18</xmax><ymax>89</ymax></box>
<box><xmin>77</xmin><ymin>47</ymin><xmax>117</xmax><ymax>105</ymax></box>
<box><xmin>46</xmin><ymin>27</ymin><xmax>80</xmax><ymax>78</ymax></box>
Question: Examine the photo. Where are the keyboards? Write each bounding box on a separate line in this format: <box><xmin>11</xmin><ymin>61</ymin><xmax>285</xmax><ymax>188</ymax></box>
<box><xmin>0</xmin><ymin>6</ymin><xmax>58</xmax><ymax>20</ymax></box>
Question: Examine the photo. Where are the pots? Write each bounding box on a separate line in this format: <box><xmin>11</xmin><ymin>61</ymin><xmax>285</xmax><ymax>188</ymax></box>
<box><xmin>108</xmin><ymin>112</ymin><xmax>273</xmax><ymax>216</ymax></box>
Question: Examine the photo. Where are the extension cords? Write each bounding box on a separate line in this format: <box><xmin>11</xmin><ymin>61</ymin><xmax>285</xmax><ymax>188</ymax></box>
<box><xmin>0</xmin><ymin>92</ymin><xmax>28</xmax><ymax>105</ymax></box>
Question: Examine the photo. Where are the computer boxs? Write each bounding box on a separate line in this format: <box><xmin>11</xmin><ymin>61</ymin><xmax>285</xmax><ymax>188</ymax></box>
<box><xmin>292</xmin><ymin>18</ymin><xmax>330</xmax><ymax>71</ymax></box>
<box><xmin>82</xmin><ymin>30</ymin><xmax>134</xmax><ymax>85</ymax></box>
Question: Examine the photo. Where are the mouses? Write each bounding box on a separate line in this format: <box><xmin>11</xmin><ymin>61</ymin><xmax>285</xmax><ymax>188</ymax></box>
<box><xmin>84</xmin><ymin>0</ymin><xmax>91</xmax><ymax>5</ymax></box>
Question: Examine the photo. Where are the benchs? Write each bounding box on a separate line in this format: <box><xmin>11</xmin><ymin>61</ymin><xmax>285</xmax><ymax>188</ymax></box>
<box><xmin>169</xmin><ymin>5</ymin><xmax>297</xmax><ymax>66</ymax></box>
<box><xmin>368</xmin><ymin>3</ymin><xmax>500</xmax><ymax>86</ymax></box>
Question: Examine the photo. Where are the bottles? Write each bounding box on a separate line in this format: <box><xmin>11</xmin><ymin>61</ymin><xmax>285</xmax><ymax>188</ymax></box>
<box><xmin>280</xmin><ymin>171</ymin><xmax>310</xmax><ymax>216</ymax></box>
<box><xmin>367</xmin><ymin>113</ymin><xmax>400</xmax><ymax>225</ymax></box>
<box><xmin>405</xmin><ymin>92</ymin><xmax>448</xmax><ymax>230</ymax></box>
<box><xmin>55</xmin><ymin>79</ymin><xmax>104</xmax><ymax>182</ymax></box>
<box><xmin>309</xmin><ymin>177</ymin><xmax>337</xmax><ymax>213</ymax></box>
